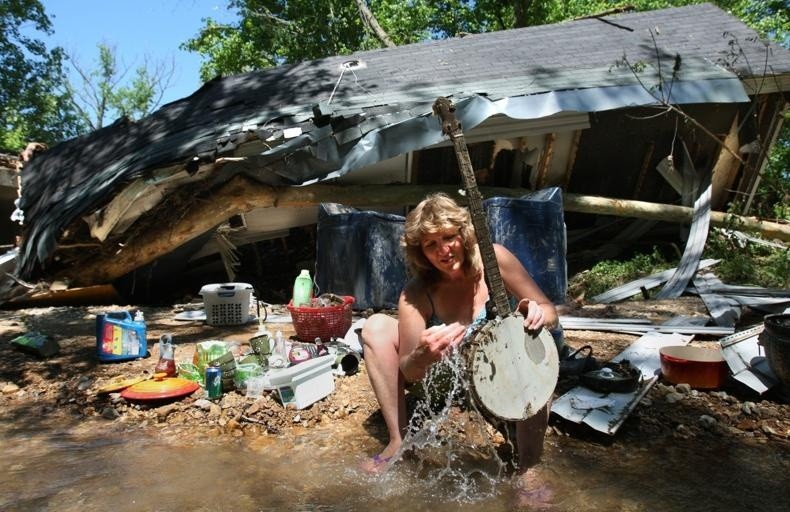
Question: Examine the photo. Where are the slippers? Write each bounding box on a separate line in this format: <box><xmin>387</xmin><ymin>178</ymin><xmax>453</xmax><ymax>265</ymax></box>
<box><xmin>359</xmin><ymin>453</ymin><xmax>410</xmax><ymax>472</ymax></box>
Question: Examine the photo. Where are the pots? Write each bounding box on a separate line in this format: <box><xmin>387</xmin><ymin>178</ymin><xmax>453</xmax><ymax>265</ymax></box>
<box><xmin>659</xmin><ymin>345</ymin><xmax>731</xmax><ymax>390</ymax></box>
<box><xmin>579</xmin><ymin>363</ymin><xmax>642</xmax><ymax>393</ymax></box>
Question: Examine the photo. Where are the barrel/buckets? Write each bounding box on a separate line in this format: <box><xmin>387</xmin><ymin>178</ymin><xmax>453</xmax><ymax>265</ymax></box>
<box><xmin>97</xmin><ymin>308</ymin><xmax>146</xmax><ymax>362</ymax></box>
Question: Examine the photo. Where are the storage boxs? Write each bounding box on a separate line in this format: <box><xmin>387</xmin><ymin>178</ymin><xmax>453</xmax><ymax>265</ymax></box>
<box><xmin>199</xmin><ymin>283</ymin><xmax>255</xmax><ymax>326</ymax></box>
<box><xmin>270</xmin><ymin>356</ymin><xmax>337</xmax><ymax>412</ymax></box>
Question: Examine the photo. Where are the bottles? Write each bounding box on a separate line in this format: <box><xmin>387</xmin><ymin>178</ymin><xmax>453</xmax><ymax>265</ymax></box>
<box><xmin>155</xmin><ymin>334</ymin><xmax>177</xmax><ymax>377</ymax></box>
<box><xmin>315</xmin><ymin>336</ymin><xmax>328</xmax><ymax>357</ymax></box>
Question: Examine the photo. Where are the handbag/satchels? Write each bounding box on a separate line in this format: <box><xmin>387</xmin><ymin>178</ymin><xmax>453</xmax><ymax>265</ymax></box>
<box><xmin>556</xmin><ymin>346</ymin><xmax>643</xmax><ymax>397</ymax></box>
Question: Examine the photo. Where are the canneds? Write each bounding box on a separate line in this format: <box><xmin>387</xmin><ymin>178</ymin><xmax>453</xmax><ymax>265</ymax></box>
<box><xmin>206</xmin><ymin>367</ymin><xmax>223</xmax><ymax>399</ymax></box>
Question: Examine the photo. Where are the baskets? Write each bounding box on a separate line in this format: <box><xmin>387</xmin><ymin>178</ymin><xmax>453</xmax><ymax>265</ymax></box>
<box><xmin>288</xmin><ymin>296</ymin><xmax>355</xmax><ymax>343</ymax></box>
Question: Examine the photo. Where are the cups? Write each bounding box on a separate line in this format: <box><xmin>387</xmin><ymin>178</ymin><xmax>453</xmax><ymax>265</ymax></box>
<box><xmin>249</xmin><ymin>334</ymin><xmax>277</xmax><ymax>355</ymax></box>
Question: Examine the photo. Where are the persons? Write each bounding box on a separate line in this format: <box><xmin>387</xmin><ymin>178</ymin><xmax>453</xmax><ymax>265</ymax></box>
<box><xmin>358</xmin><ymin>193</ymin><xmax>560</xmax><ymax>510</ymax></box>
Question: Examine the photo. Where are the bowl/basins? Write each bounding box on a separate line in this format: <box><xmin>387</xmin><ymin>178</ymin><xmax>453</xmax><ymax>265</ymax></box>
<box><xmin>208</xmin><ymin>350</ymin><xmax>237</xmax><ymax>388</ymax></box>
